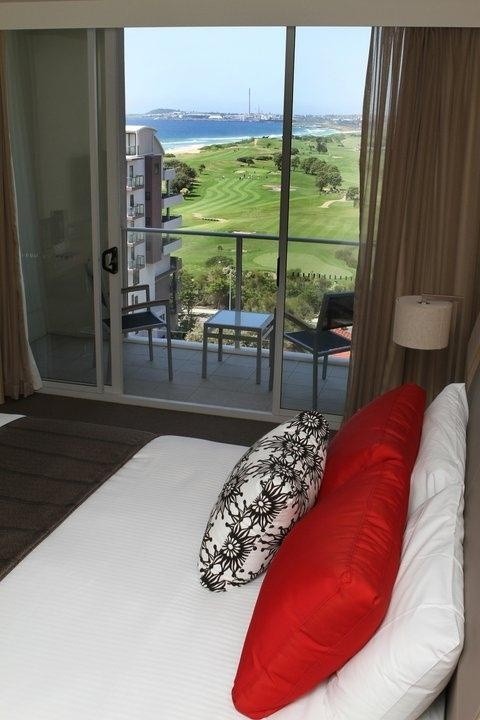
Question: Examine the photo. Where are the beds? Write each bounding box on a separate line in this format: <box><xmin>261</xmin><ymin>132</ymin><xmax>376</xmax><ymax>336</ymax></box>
<box><xmin>1</xmin><ymin>365</ymin><xmax>480</xmax><ymax>719</ymax></box>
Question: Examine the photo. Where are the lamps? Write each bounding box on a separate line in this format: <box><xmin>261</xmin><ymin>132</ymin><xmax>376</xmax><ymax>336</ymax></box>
<box><xmin>392</xmin><ymin>293</ymin><xmax>465</xmax><ymax>384</ymax></box>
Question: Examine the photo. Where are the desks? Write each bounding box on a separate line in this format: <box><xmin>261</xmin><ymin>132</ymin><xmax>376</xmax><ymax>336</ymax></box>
<box><xmin>202</xmin><ymin>310</ymin><xmax>275</xmax><ymax>385</ymax></box>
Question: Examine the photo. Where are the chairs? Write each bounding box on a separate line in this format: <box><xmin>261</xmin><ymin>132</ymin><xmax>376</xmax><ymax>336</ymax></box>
<box><xmin>269</xmin><ymin>293</ymin><xmax>354</xmax><ymax>410</ymax></box>
<box><xmin>89</xmin><ymin>274</ymin><xmax>174</xmax><ymax>381</ymax></box>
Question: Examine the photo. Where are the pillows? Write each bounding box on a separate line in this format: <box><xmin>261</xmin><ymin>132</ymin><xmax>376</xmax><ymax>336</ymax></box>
<box><xmin>198</xmin><ymin>410</ymin><xmax>330</xmax><ymax>593</ymax></box>
<box><xmin>232</xmin><ymin>459</ymin><xmax>411</xmax><ymax>718</ymax></box>
<box><xmin>321</xmin><ymin>482</ymin><xmax>467</xmax><ymax>718</ymax></box>
<box><xmin>405</xmin><ymin>384</ymin><xmax>468</xmax><ymax>521</ymax></box>
